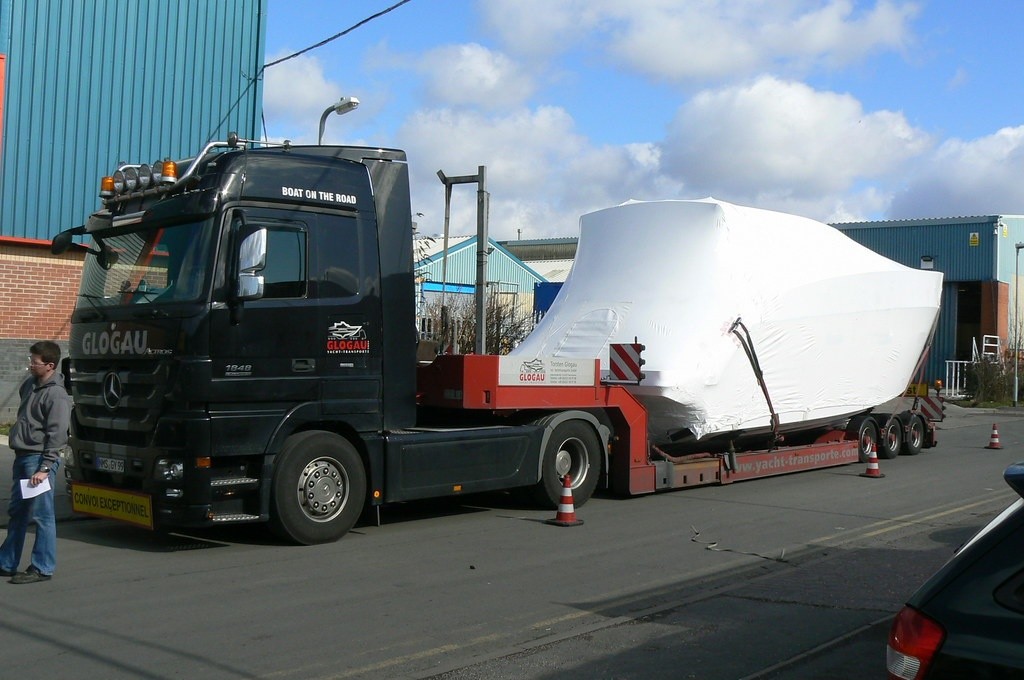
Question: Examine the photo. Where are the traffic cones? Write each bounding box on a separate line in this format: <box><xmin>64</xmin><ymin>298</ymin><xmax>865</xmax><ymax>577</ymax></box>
<box><xmin>546</xmin><ymin>476</ymin><xmax>583</xmax><ymax>527</ymax></box>
<box><xmin>985</xmin><ymin>425</ymin><xmax>1003</xmax><ymax>450</ymax></box>
<box><xmin>858</xmin><ymin>442</ymin><xmax>886</xmax><ymax>478</ymax></box>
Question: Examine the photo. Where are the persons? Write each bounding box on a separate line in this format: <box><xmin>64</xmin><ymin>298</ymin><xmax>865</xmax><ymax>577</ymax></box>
<box><xmin>0</xmin><ymin>340</ymin><xmax>72</xmax><ymax>584</ymax></box>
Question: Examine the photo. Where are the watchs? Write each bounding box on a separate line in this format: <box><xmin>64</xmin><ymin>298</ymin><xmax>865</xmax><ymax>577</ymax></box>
<box><xmin>40</xmin><ymin>467</ymin><xmax>50</xmax><ymax>472</ymax></box>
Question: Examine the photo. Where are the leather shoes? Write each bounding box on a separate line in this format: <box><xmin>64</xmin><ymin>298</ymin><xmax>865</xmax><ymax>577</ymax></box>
<box><xmin>12</xmin><ymin>564</ymin><xmax>51</xmax><ymax>583</ymax></box>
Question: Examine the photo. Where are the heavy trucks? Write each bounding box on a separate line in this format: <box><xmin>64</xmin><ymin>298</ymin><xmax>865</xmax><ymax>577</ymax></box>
<box><xmin>50</xmin><ymin>136</ymin><xmax>947</xmax><ymax>547</ymax></box>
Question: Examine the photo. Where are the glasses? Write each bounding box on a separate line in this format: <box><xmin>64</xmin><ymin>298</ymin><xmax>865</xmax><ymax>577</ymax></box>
<box><xmin>27</xmin><ymin>355</ymin><xmax>48</xmax><ymax>366</ymax></box>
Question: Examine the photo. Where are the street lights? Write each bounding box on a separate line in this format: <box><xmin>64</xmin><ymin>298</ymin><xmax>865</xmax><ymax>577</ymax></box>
<box><xmin>1013</xmin><ymin>243</ymin><xmax>1024</xmax><ymax>406</ymax></box>
<box><xmin>318</xmin><ymin>98</ymin><xmax>360</xmax><ymax>145</ymax></box>
